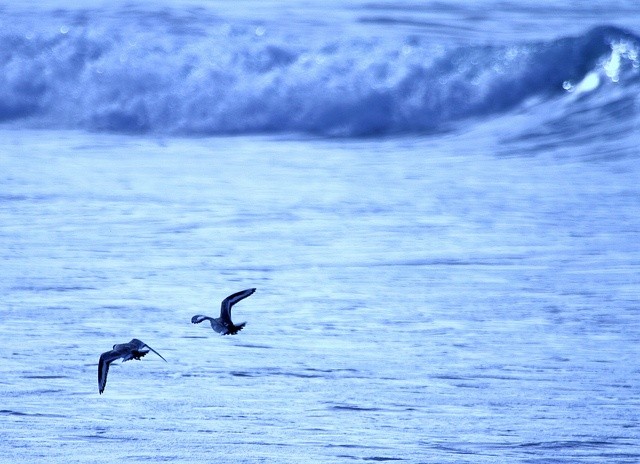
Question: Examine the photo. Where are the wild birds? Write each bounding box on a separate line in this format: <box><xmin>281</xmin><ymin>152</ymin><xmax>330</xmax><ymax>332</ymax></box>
<box><xmin>191</xmin><ymin>288</ymin><xmax>256</xmax><ymax>335</ymax></box>
<box><xmin>99</xmin><ymin>338</ymin><xmax>167</xmax><ymax>395</ymax></box>
